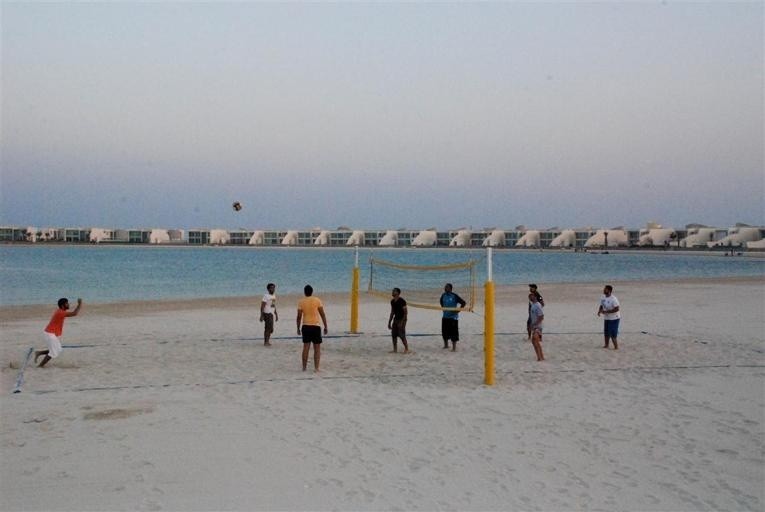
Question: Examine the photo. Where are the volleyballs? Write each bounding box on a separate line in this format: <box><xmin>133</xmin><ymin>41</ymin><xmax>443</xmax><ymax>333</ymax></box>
<box><xmin>233</xmin><ymin>202</ymin><xmax>241</xmax><ymax>210</ymax></box>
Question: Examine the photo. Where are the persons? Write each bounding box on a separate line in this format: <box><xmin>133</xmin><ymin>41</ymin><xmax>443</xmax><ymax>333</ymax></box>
<box><xmin>526</xmin><ymin>282</ymin><xmax>546</xmax><ymax>342</ymax></box>
<box><xmin>296</xmin><ymin>283</ymin><xmax>328</xmax><ymax>373</ymax></box>
<box><xmin>528</xmin><ymin>290</ymin><xmax>545</xmax><ymax>361</ymax></box>
<box><xmin>388</xmin><ymin>287</ymin><xmax>410</xmax><ymax>353</ymax></box>
<box><xmin>597</xmin><ymin>284</ymin><xmax>621</xmax><ymax>350</ymax></box>
<box><xmin>260</xmin><ymin>283</ymin><xmax>279</xmax><ymax>347</ymax></box>
<box><xmin>440</xmin><ymin>283</ymin><xmax>467</xmax><ymax>353</ymax></box>
<box><xmin>34</xmin><ymin>297</ymin><xmax>82</xmax><ymax>368</ymax></box>
